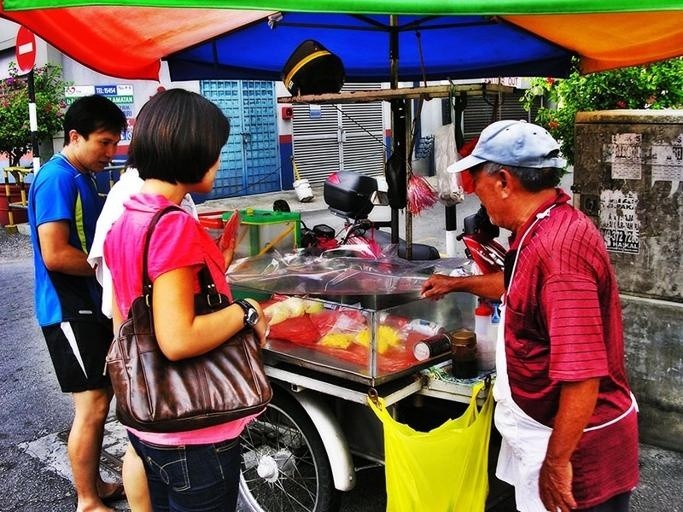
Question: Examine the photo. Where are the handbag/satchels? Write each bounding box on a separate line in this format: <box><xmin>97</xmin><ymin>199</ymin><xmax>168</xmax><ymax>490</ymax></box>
<box><xmin>104</xmin><ymin>206</ymin><xmax>277</xmax><ymax>435</ymax></box>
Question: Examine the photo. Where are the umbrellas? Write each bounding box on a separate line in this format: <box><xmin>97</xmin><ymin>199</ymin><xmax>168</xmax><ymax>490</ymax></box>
<box><xmin>0</xmin><ymin>0</ymin><xmax>683</xmax><ymax>266</ymax></box>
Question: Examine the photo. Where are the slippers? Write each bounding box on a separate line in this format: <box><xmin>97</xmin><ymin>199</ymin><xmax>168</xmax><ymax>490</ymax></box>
<box><xmin>71</xmin><ymin>482</ymin><xmax>129</xmax><ymax>505</ymax></box>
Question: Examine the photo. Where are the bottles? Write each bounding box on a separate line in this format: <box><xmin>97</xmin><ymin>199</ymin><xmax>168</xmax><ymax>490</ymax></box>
<box><xmin>411</xmin><ymin>299</ymin><xmax>496</xmax><ymax>379</ymax></box>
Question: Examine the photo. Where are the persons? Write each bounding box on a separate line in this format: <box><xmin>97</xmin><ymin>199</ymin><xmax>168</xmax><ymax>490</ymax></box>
<box><xmin>26</xmin><ymin>94</ymin><xmax>130</xmax><ymax>512</ymax></box>
<box><xmin>100</xmin><ymin>87</ymin><xmax>271</xmax><ymax>511</ymax></box>
<box><xmin>84</xmin><ymin>158</ymin><xmax>201</xmax><ymax>512</ymax></box>
<box><xmin>418</xmin><ymin>118</ymin><xmax>641</xmax><ymax>511</ymax></box>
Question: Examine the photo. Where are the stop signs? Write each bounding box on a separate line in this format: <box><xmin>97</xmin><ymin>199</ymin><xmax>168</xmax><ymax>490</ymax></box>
<box><xmin>16</xmin><ymin>24</ymin><xmax>37</xmax><ymax>70</ymax></box>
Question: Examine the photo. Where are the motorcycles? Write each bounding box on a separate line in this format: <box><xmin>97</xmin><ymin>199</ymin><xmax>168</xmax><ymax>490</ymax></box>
<box><xmin>325</xmin><ymin>171</ymin><xmax>507</xmax><ymax>278</ymax></box>
<box><xmin>275</xmin><ymin>197</ymin><xmax>392</xmax><ymax>274</ymax></box>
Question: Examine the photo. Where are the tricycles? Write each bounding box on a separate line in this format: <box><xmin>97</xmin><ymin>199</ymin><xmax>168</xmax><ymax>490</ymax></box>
<box><xmin>236</xmin><ymin>366</ymin><xmax>497</xmax><ymax>512</ymax></box>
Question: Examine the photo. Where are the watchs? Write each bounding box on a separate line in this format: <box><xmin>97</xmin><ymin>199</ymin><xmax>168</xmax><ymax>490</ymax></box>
<box><xmin>233</xmin><ymin>299</ymin><xmax>260</xmax><ymax>328</ymax></box>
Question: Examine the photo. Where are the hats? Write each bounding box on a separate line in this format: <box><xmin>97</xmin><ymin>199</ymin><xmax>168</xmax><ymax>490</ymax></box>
<box><xmin>445</xmin><ymin>117</ymin><xmax>570</xmax><ymax>176</ymax></box>
